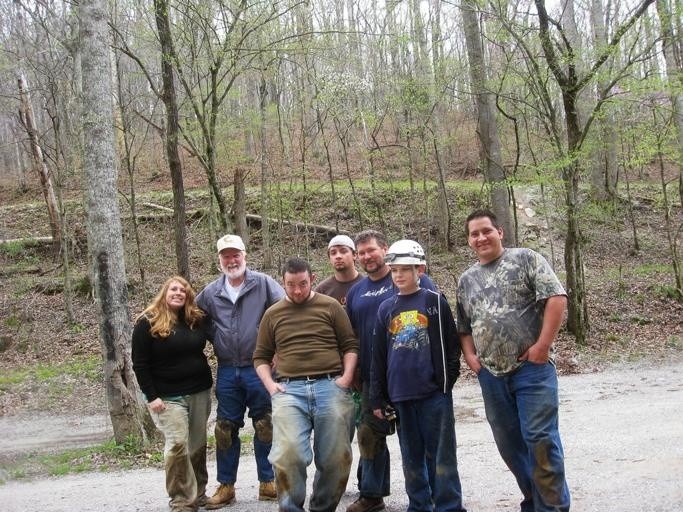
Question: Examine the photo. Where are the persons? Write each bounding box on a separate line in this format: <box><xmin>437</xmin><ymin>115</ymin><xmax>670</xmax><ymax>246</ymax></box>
<box><xmin>345</xmin><ymin>229</ymin><xmax>436</xmax><ymax>512</ymax></box>
<box><xmin>313</xmin><ymin>234</ymin><xmax>391</xmax><ymax>497</ymax></box>
<box><xmin>131</xmin><ymin>275</ymin><xmax>215</xmax><ymax>512</ymax></box>
<box><xmin>455</xmin><ymin>209</ymin><xmax>571</xmax><ymax>512</ymax></box>
<box><xmin>368</xmin><ymin>238</ymin><xmax>463</xmax><ymax>512</ymax></box>
<box><xmin>191</xmin><ymin>234</ymin><xmax>288</xmax><ymax>509</ymax></box>
<box><xmin>251</xmin><ymin>258</ymin><xmax>360</xmax><ymax>512</ymax></box>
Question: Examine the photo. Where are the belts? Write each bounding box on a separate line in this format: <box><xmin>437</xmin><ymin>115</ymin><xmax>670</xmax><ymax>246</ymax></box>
<box><xmin>276</xmin><ymin>370</ymin><xmax>342</xmax><ymax>383</ymax></box>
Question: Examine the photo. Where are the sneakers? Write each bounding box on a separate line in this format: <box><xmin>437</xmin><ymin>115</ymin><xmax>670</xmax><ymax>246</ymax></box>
<box><xmin>345</xmin><ymin>493</ymin><xmax>385</xmax><ymax>512</ymax></box>
<box><xmin>257</xmin><ymin>480</ymin><xmax>279</xmax><ymax>501</ymax></box>
<box><xmin>202</xmin><ymin>483</ymin><xmax>235</xmax><ymax>510</ymax></box>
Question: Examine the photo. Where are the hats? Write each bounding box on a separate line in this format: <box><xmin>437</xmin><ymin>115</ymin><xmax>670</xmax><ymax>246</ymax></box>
<box><xmin>327</xmin><ymin>234</ymin><xmax>355</xmax><ymax>253</ymax></box>
<box><xmin>216</xmin><ymin>234</ymin><xmax>245</xmax><ymax>252</ymax></box>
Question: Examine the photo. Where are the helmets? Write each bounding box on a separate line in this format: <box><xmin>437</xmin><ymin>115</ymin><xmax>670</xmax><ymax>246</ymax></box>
<box><xmin>384</xmin><ymin>239</ymin><xmax>426</xmax><ymax>265</ymax></box>
